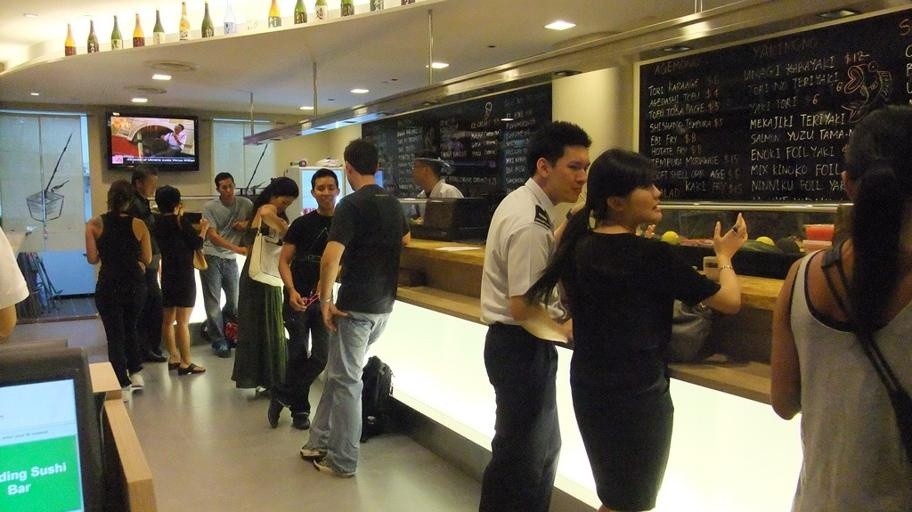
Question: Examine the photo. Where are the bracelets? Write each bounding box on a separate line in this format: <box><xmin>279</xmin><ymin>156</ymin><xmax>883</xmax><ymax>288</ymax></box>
<box><xmin>719</xmin><ymin>266</ymin><xmax>734</xmax><ymax>271</ymax></box>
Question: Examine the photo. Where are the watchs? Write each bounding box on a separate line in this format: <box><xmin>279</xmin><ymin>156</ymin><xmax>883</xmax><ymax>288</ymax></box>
<box><xmin>319</xmin><ymin>298</ymin><xmax>332</xmax><ymax>303</ymax></box>
<box><xmin>566</xmin><ymin>208</ymin><xmax>574</xmax><ymax>220</ymax></box>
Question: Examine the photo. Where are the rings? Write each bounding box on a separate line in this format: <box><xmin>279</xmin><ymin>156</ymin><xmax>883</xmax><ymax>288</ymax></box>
<box><xmin>731</xmin><ymin>225</ymin><xmax>738</xmax><ymax>234</ymax></box>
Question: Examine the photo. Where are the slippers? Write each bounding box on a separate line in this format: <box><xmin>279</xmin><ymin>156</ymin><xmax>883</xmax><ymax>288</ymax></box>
<box><xmin>177</xmin><ymin>363</ymin><xmax>203</xmax><ymax>375</ymax></box>
<box><xmin>167</xmin><ymin>357</ymin><xmax>182</xmax><ymax>371</ymax></box>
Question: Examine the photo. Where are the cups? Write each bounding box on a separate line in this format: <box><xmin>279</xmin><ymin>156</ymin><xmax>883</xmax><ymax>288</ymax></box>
<box><xmin>702</xmin><ymin>255</ymin><xmax>724</xmax><ymax>281</ymax></box>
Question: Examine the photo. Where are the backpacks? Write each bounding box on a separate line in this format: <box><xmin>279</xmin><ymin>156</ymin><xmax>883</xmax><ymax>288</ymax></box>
<box><xmin>360</xmin><ymin>356</ymin><xmax>393</xmax><ymax>443</ymax></box>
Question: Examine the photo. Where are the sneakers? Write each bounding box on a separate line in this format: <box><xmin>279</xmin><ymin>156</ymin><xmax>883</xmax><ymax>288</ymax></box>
<box><xmin>217</xmin><ymin>343</ymin><xmax>231</xmax><ymax>357</ymax></box>
<box><xmin>301</xmin><ymin>444</ymin><xmax>325</xmax><ymax>459</ymax></box>
<box><xmin>313</xmin><ymin>461</ymin><xmax>357</xmax><ymax>477</ymax></box>
<box><xmin>120</xmin><ymin>386</ymin><xmax>130</xmax><ymax>401</ymax></box>
<box><xmin>131</xmin><ymin>374</ymin><xmax>144</xmax><ymax>390</ymax></box>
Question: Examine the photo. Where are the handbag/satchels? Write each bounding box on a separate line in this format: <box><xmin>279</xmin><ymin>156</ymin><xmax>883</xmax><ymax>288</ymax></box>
<box><xmin>249</xmin><ymin>205</ymin><xmax>288</xmax><ymax>288</ymax></box>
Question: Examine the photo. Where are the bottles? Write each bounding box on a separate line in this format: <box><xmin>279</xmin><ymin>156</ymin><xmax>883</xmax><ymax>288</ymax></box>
<box><xmin>112</xmin><ymin>15</ymin><xmax>122</xmax><ymax>48</ymax></box>
<box><xmin>202</xmin><ymin>3</ymin><xmax>213</xmax><ymax>38</ymax></box>
<box><xmin>268</xmin><ymin>1</ymin><xmax>281</xmax><ymax>28</ymax></box>
<box><xmin>134</xmin><ymin>15</ymin><xmax>144</xmax><ymax>47</ymax></box>
<box><xmin>181</xmin><ymin>4</ymin><xmax>189</xmax><ymax>41</ymax></box>
<box><xmin>224</xmin><ymin>0</ymin><xmax>235</xmax><ymax>34</ymax></box>
<box><xmin>342</xmin><ymin>0</ymin><xmax>353</xmax><ymax>15</ymax></box>
<box><xmin>402</xmin><ymin>0</ymin><xmax>414</xmax><ymax>5</ymax></box>
<box><xmin>315</xmin><ymin>1</ymin><xmax>327</xmax><ymax>19</ymax></box>
<box><xmin>154</xmin><ymin>10</ymin><xmax>163</xmax><ymax>44</ymax></box>
<box><xmin>370</xmin><ymin>0</ymin><xmax>384</xmax><ymax>11</ymax></box>
<box><xmin>294</xmin><ymin>1</ymin><xmax>304</xmax><ymax>23</ymax></box>
<box><xmin>88</xmin><ymin>21</ymin><xmax>99</xmax><ymax>53</ymax></box>
<box><xmin>65</xmin><ymin>25</ymin><xmax>76</xmax><ymax>57</ymax></box>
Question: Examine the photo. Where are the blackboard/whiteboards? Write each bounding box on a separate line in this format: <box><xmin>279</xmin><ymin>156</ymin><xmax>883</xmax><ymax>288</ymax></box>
<box><xmin>361</xmin><ymin>81</ymin><xmax>553</xmax><ymax>198</ymax></box>
<box><xmin>634</xmin><ymin>4</ymin><xmax>912</xmax><ymax>212</ymax></box>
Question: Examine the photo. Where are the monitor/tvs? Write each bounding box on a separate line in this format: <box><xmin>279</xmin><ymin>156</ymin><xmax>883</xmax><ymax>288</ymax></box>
<box><xmin>1</xmin><ymin>347</ymin><xmax>105</xmax><ymax>512</ymax></box>
<box><xmin>105</xmin><ymin>111</ymin><xmax>201</xmax><ymax>172</ymax></box>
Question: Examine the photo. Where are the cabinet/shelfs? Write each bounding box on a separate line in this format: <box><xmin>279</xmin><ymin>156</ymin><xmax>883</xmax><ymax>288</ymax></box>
<box><xmin>157</xmin><ymin>198</ymin><xmax>844</xmax><ymax>512</ymax></box>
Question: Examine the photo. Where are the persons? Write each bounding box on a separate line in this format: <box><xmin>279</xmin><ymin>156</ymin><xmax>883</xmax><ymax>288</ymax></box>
<box><xmin>771</xmin><ymin>105</ymin><xmax>912</xmax><ymax>512</ymax></box>
<box><xmin>152</xmin><ymin>184</ymin><xmax>210</xmax><ymax>375</ymax></box>
<box><xmin>85</xmin><ymin>180</ymin><xmax>151</xmax><ymax>404</ymax></box>
<box><xmin>0</xmin><ymin>226</ymin><xmax>29</xmax><ymax>338</ymax></box>
<box><xmin>524</xmin><ymin>149</ymin><xmax>748</xmax><ymax>512</ymax></box>
<box><xmin>232</xmin><ymin>176</ymin><xmax>299</xmax><ymax>396</ymax></box>
<box><xmin>298</xmin><ymin>139</ymin><xmax>410</xmax><ymax>479</ymax></box>
<box><xmin>201</xmin><ymin>172</ymin><xmax>254</xmax><ymax>359</ymax></box>
<box><xmin>150</xmin><ymin>125</ymin><xmax>188</xmax><ymax>158</ymax></box>
<box><xmin>479</xmin><ymin>122</ymin><xmax>592</xmax><ymax>512</ymax></box>
<box><xmin>267</xmin><ymin>168</ymin><xmax>340</xmax><ymax>430</ymax></box>
<box><xmin>127</xmin><ymin>169</ymin><xmax>168</xmax><ymax>362</ymax></box>
<box><xmin>410</xmin><ymin>150</ymin><xmax>465</xmax><ymax>200</ymax></box>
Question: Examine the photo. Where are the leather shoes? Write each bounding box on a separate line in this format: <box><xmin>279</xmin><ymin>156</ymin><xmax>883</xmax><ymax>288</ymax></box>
<box><xmin>268</xmin><ymin>385</ymin><xmax>282</xmax><ymax>428</ymax></box>
<box><xmin>293</xmin><ymin>413</ymin><xmax>310</xmax><ymax>428</ymax></box>
